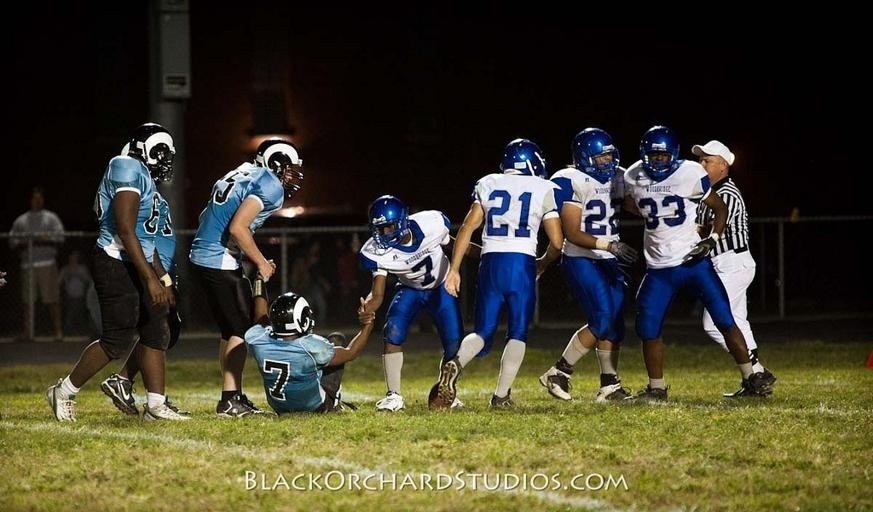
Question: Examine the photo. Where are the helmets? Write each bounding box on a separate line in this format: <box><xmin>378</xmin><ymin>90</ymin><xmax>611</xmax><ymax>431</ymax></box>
<box><xmin>126</xmin><ymin>119</ymin><xmax>177</xmax><ymax>187</ymax></box>
<box><xmin>252</xmin><ymin>139</ymin><xmax>305</xmax><ymax>200</ymax></box>
<box><xmin>267</xmin><ymin>290</ymin><xmax>312</xmax><ymax>338</ymax></box>
<box><xmin>639</xmin><ymin>124</ymin><xmax>677</xmax><ymax>181</ymax></box>
<box><xmin>367</xmin><ymin>194</ymin><xmax>402</xmax><ymax>246</ymax></box>
<box><xmin>495</xmin><ymin>138</ymin><xmax>548</xmax><ymax>181</ymax></box>
<box><xmin>569</xmin><ymin>127</ymin><xmax>618</xmax><ymax>182</ymax></box>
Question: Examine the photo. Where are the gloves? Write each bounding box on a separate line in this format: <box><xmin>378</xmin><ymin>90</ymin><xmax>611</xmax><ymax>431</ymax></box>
<box><xmin>687</xmin><ymin>232</ymin><xmax>719</xmax><ymax>263</ymax></box>
<box><xmin>606</xmin><ymin>237</ymin><xmax>640</xmax><ymax>268</ymax></box>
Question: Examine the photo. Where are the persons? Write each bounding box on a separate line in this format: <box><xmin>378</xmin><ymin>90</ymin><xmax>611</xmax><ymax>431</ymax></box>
<box><xmin>59</xmin><ymin>245</ymin><xmax>95</xmax><ymax>335</ymax></box>
<box><xmin>334</xmin><ymin>237</ymin><xmax>361</xmax><ymax>321</ymax></box>
<box><xmin>308</xmin><ymin>245</ymin><xmax>331</xmax><ymax>326</ymax></box>
<box><xmin>690</xmin><ymin>138</ymin><xmax>778</xmax><ymax>395</ymax></box>
<box><xmin>429</xmin><ymin>137</ymin><xmax>563</xmax><ymax>411</ymax></box>
<box><xmin>538</xmin><ymin>126</ymin><xmax>637</xmax><ymax>405</ymax></box>
<box><xmin>187</xmin><ymin>142</ymin><xmax>303</xmax><ymax>417</ymax></box>
<box><xmin>289</xmin><ymin>240</ymin><xmax>319</xmax><ymax>300</ymax></box>
<box><xmin>624</xmin><ymin>123</ymin><xmax>773</xmax><ymax>400</ymax></box>
<box><xmin>358</xmin><ymin>194</ymin><xmax>481</xmax><ymax>410</ymax></box>
<box><xmin>244</xmin><ymin>259</ymin><xmax>374</xmax><ymax>415</ymax></box>
<box><xmin>8</xmin><ymin>186</ymin><xmax>64</xmax><ymax>343</ymax></box>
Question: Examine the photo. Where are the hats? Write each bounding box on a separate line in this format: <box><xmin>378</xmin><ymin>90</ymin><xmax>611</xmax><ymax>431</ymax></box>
<box><xmin>691</xmin><ymin>140</ymin><xmax>735</xmax><ymax>168</ymax></box>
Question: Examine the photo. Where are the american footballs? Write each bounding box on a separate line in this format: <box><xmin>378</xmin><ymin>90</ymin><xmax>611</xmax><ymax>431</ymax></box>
<box><xmin>428</xmin><ymin>382</ymin><xmax>456</xmax><ymax>412</ymax></box>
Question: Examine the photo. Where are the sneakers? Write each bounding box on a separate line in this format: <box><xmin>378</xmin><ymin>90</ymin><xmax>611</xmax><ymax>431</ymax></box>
<box><xmin>428</xmin><ymin>354</ymin><xmax>463</xmax><ymax>417</ymax></box>
<box><xmin>100</xmin><ymin>370</ymin><xmax>140</xmax><ymax>417</ymax></box>
<box><xmin>42</xmin><ymin>376</ymin><xmax>79</xmax><ymax>424</ymax></box>
<box><xmin>214</xmin><ymin>388</ymin><xmax>278</xmax><ymax>423</ymax></box>
<box><xmin>489</xmin><ymin>387</ymin><xmax>520</xmax><ymax>411</ymax></box>
<box><xmin>622</xmin><ymin>383</ymin><xmax>672</xmax><ymax>407</ymax></box>
<box><xmin>595</xmin><ymin>373</ymin><xmax>633</xmax><ymax>403</ymax></box>
<box><xmin>375</xmin><ymin>391</ymin><xmax>406</xmax><ymax>413</ymax></box>
<box><xmin>144</xmin><ymin>398</ymin><xmax>193</xmax><ymax>423</ymax></box>
<box><xmin>721</xmin><ymin>371</ymin><xmax>773</xmax><ymax>397</ymax></box>
<box><xmin>761</xmin><ymin>367</ymin><xmax>777</xmax><ymax>384</ymax></box>
<box><xmin>449</xmin><ymin>396</ymin><xmax>466</xmax><ymax>410</ymax></box>
<box><xmin>538</xmin><ymin>362</ymin><xmax>575</xmax><ymax>402</ymax></box>
